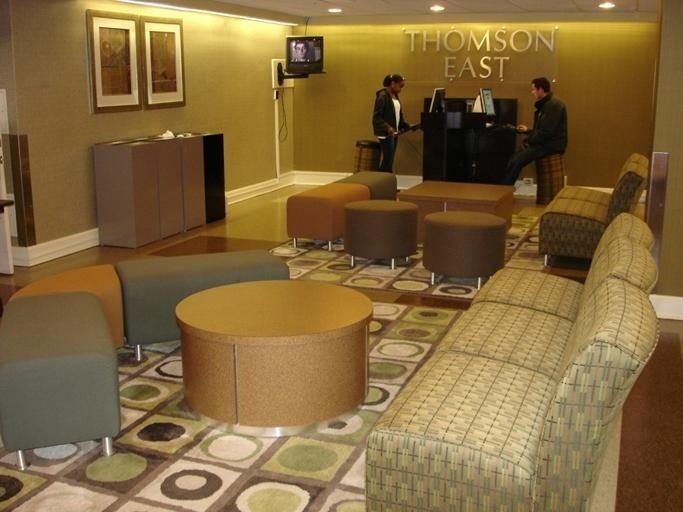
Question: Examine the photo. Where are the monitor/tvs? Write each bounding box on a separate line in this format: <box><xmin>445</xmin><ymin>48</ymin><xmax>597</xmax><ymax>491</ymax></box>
<box><xmin>479</xmin><ymin>88</ymin><xmax>496</xmax><ymax>115</ymax></box>
<box><xmin>429</xmin><ymin>88</ymin><xmax>446</xmax><ymax>112</ymax></box>
<box><xmin>285</xmin><ymin>36</ymin><xmax>323</xmax><ymax>74</ymax></box>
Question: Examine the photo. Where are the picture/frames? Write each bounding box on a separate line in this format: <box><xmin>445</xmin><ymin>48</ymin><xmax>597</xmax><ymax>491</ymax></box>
<box><xmin>86</xmin><ymin>9</ymin><xmax>143</xmax><ymax>114</ymax></box>
<box><xmin>139</xmin><ymin>15</ymin><xmax>187</xmax><ymax>110</ymax></box>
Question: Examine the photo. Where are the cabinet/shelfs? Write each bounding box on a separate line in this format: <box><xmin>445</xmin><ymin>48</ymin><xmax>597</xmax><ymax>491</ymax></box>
<box><xmin>92</xmin><ymin>131</ymin><xmax>226</xmax><ymax>249</ymax></box>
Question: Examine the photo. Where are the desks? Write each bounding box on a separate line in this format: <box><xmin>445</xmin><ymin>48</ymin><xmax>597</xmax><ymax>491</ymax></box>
<box><xmin>420</xmin><ymin>98</ymin><xmax>518</xmax><ymax>188</ymax></box>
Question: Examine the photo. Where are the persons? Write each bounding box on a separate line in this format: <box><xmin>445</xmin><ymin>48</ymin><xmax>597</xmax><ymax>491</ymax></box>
<box><xmin>371</xmin><ymin>74</ymin><xmax>417</xmax><ymax>173</ymax></box>
<box><xmin>291</xmin><ymin>40</ymin><xmax>313</xmax><ymax>63</ymax></box>
<box><xmin>497</xmin><ymin>76</ymin><xmax>568</xmax><ymax>185</ymax></box>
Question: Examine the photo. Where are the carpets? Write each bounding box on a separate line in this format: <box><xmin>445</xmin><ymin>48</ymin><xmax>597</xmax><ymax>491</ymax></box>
<box><xmin>0</xmin><ymin>214</ymin><xmax>623</xmax><ymax>512</ymax></box>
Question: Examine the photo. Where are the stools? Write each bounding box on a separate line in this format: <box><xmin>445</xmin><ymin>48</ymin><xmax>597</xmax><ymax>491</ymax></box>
<box><xmin>7</xmin><ymin>264</ymin><xmax>124</xmax><ymax>350</ymax></box>
<box><xmin>535</xmin><ymin>151</ymin><xmax>565</xmax><ymax>205</ymax></box>
<box><xmin>0</xmin><ymin>291</ymin><xmax>121</xmax><ymax>471</ymax></box>
<box><xmin>287</xmin><ymin>171</ymin><xmax>507</xmax><ymax>291</ymax></box>
<box><xmin>116</xmin><ymin>249</ymin><xmax>289</xmax><ymax>362</ymax></box>
<box><xmin>353</xmin><ymin>140</ymin><xmax>381</xmax><ymax>174</ymax></box>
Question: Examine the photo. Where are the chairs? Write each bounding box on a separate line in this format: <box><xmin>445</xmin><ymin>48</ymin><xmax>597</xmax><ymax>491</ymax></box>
<box><xmin>538</xmin><ymin>153</ymin><xmax>650</xmax><ymax>267</ymax></box>
